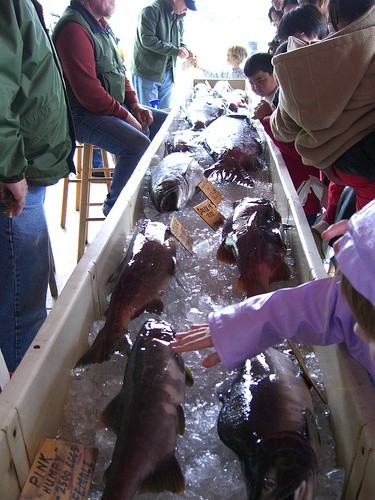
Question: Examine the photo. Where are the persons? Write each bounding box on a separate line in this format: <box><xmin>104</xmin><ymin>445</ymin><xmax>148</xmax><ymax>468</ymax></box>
<box><xmin>92</xmin><ymin>143</ymin><xmax>114</xmax><ymax>177</ymax></box>
<box><xmin>243</xmin><ymin>0</ymin><xmax>375</xmax><ymax>281</ymax></box>
<box><xmin>0</xmin><ymin>0</ymin><xmax>79</xmax><ymax>376</ymax></box>
<box><xmin>191</xmin><ymin>46</ymin><xmax>247</xmax><ymax>79</ymax></box>
<box><xmin>131</xmin><ymin>0</ymin><xmax>198</xmax><ymax>114</ymax></box>
<box><xmin>51</xmin><ymin>0</ymin><xmax>170</xmax><ymax>216</ymax></box>
<box><xmin>172</xmin><ymin>193</ymin><xmax>375</xmax><ymax>381</ymax></box>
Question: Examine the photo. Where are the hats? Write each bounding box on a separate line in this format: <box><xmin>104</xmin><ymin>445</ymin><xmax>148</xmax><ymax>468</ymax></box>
<box><xmin>184</xmin><ymin>0</ymin><xmax>197</xmax><ymax>11</ymax></box>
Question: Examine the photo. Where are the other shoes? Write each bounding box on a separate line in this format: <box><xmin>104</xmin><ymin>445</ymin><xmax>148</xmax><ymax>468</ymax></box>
<box><xmin>92</xmin><ymin>171</ymin><xmax>115</xmax><ymax>177</ymax></box>
<box><xmin>311</xmin><ymin>221</ymin><xmax>329</xmax><ymax>260</ymax></box>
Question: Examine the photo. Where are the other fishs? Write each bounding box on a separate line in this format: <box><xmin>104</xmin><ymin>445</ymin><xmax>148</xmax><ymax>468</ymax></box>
<box><xmin>97</xmin><ymin>317</ymin><xmax>193</xmax><ymax>500</ymax></box>
<box><xmin>216</xmin><ymin>345</ymin><xmax>322</xmax><ymax>500</ymax></box>
<box><xmin>149</xmin><ymin>80</ymin><xmax>264</xmax><ymax>214</ymax></box>
<box><xmin>216</xmin><ymin>196</ymin><xmax>292</xmax><ymax>300</ymax></box>
<box><xmin>71</xmin><ymin>219</ymin><xmax>188</xmax><ymax>371</ymax></box>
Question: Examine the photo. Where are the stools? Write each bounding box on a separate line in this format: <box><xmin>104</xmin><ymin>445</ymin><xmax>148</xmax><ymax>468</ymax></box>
<box><xmin>60</xmin><ymin>134</ymin><xmax>119</xmax><ymax>265</ymax></box>
